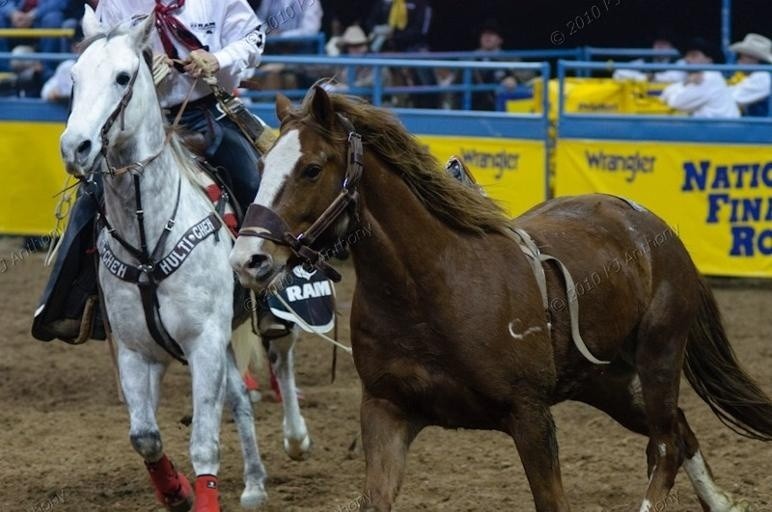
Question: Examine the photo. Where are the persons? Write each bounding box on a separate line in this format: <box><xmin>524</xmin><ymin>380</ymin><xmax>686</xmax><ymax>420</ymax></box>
<box><xmin>33</xmin><ymin>0</ymin><xmax>294</xmax><ymax>344</ymax></box>
<box><xmin>0</xmin><ymin>0</ymin><xmax>771</xmax><ymax>121</ymax></box>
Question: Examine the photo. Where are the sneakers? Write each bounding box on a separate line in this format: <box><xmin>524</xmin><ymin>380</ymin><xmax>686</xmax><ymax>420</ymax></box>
<box><xmin>43</xmin><ymin>318</ymin><xmax>107</xmax><ymax>341</ymax></box>
<box><xmin>260</xmin><ymin>312</ymin><xmax>290</xmax><ymax>336</ymax></box>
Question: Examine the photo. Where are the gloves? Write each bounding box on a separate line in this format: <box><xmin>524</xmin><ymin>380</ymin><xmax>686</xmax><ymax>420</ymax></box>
<box><xmin>151</xmin><ymin>54</ymin><xmax>170</xmax><ymax>84</ymax></box>
<box><xmin>187</xmin><ymin>49</ymin><xmax>219</xmax><ymax>79</ymax></box>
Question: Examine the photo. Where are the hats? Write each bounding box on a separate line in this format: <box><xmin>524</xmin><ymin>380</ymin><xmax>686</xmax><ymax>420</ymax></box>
<box><xmin>729</xmin><ymin>33</ymin><xmax>771</xmax><ymax>61</ymax></box>
<box><xmin>336</xmin><ymin>26</ymin><xmax>375</xmax><ymax>49</ymax></box>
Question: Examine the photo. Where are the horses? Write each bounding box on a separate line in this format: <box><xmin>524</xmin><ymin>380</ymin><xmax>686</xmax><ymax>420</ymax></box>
<box><xmin>59</xmin><ymin>3</ymin><xmax>313</xmax><ymax>512</ymax></box>
<box><xmin>228</xmin><ymin>74</ymin><xmax>772</xmax><ymax>512</ymax></box>
